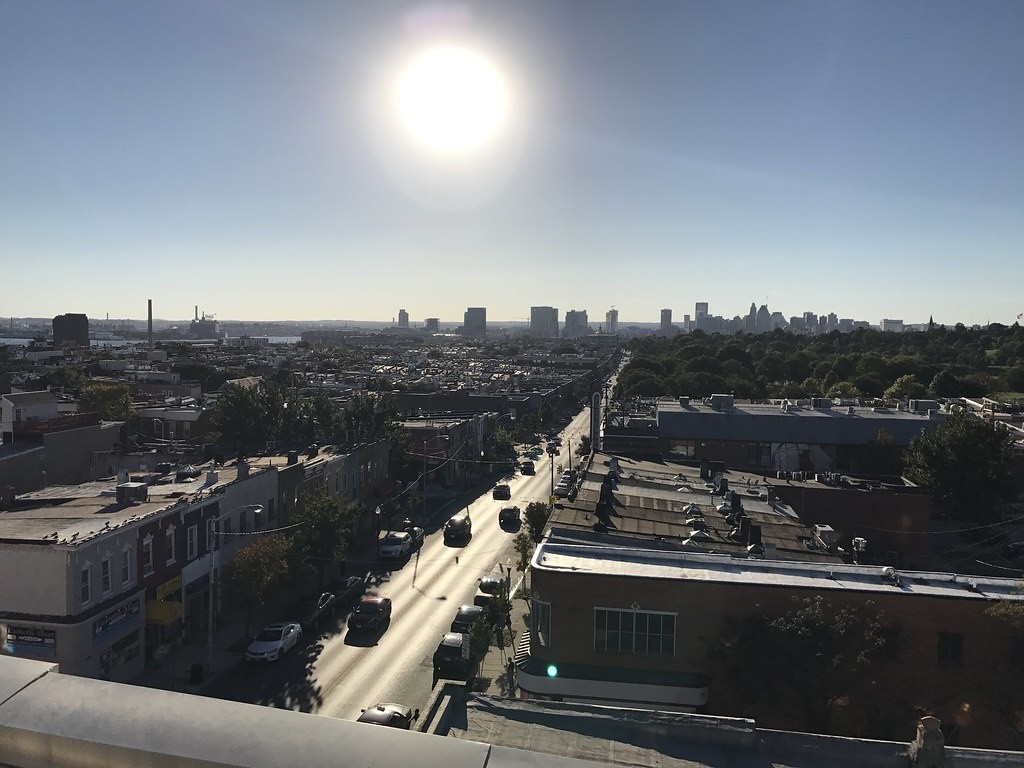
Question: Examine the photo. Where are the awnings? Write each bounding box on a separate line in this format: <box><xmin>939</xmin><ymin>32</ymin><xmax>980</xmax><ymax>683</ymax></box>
<box><xmin>146</xmin><ymin>599</ymin><xmax>182</xmax><ymax>625</ymax></box>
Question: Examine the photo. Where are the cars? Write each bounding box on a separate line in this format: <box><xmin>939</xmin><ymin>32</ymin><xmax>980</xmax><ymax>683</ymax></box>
<box><xmin>492</xmin><ymin>484</ymin><xmax>510</xmax><ymax>500</ymax></box>
<box><xmin>521</xmin><ymin>461</ymin><xmax>535</xmax><ymax>475</ymax></box>
<box><xmin>443</xmin><ymin>514</ymin><xmax>471</xmax><ymax>541</ymax></box>
<box><xmin>245</xmin><ymin>621</ymin><xmax>304</xmax><ymax>665</ymax></box>
<box><xmin>552</xmin><ymin>482</ymin><xmax>569</xmax><ymax>497</ymax></box>
<box><xmin>432</xmin><ymin>631</ymin><xmax>472</xmax><ymax>676</ymax></box>
<box><xmin>473</xmin><ymin>576</ymin><xmax>504</xmax><ymax>606</ymax></box>
<box><xmin>450</xmin><ymin>605</ymin><xmax>490</xmax><ymax>634</ymax></box>
<box><xmin>285</xmin><ymin>592</ymin><xmax>338</xmax><ymax>630</ymax></box>
<box><xmin>328</xmin><ymin>524</ymin><xmax>425</xmax><ymax>605</ymax></box>
<box><xmin>357</xmin><ymin>703</ymin><xmax>413</xmax><ymax>730</ymax></box>
<box><xmin>560</xmin><ymin>469</ymin><xmax>576</xmax><ymax>485</ymax></box>
<box><xmin>346</xmin><ymin>596</ymin><xmax>392</xmax><ymax>631</ymax></box>
<box><xmin>546</xmin><ymin>435</ymin><xmax>563</xmax><ymax>456</ymax></box>
<box><xmin>498</xmin><ymin>505</ymin><xmax>520</xmax><ymax>527</ymax></box>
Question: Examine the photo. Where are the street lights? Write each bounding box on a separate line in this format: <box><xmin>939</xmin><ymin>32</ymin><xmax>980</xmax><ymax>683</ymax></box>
<box><xmin>493</xmin><ymin>411</ymin><xmax>513</xmax><ymax>456</ymax></box>
<box><xmin>423</xmin><ymin>433</ymin><xmax>451</xmax><ymax>519</ymax></box>
<box><xmin>207</xmin><ymin>503</ymin><xmax>266</xmax><ymax>678</ymax></box>
